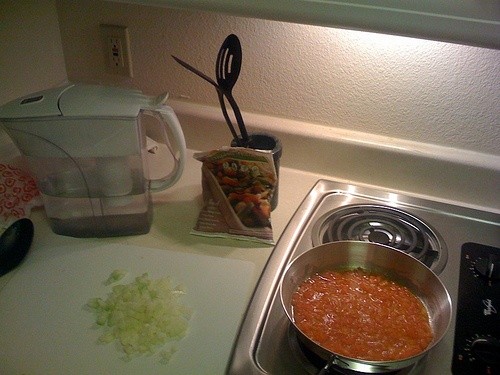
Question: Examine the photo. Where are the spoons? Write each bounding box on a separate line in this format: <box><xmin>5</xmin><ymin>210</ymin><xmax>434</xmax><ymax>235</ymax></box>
<box><xmin>0</xmin><ymin>218</ymin><xmax>34</xmax><ymax>278</ymax></box>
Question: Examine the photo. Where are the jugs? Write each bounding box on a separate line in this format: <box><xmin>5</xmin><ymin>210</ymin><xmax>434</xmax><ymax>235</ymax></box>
<box><xmin>0</xmin><ymin>83</ymin><xmax>188</xmax><ymax>239</ymax></box>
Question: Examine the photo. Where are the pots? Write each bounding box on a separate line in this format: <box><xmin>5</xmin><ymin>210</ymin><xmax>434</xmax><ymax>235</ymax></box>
<box><xmin>279</xmin><ymin>240</ymin><xmax>453</xmax><ymax>375</ymax></box>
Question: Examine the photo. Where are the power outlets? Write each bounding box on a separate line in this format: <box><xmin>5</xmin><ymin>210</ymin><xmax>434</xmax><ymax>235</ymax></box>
<box><xmin>97</xmin><ymin>22</ymin><xmax>134</xmax><ymax>81</ymax></box>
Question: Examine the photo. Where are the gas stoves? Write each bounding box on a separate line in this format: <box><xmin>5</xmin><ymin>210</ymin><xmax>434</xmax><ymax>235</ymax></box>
<box><xmin>226</xmin><ymin>178</ymin><xmax>500</xmax><ymax>375</ymax></box>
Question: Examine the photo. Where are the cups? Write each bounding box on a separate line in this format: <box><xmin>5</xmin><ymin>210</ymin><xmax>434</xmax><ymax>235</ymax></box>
<box><xmin>231</xmin><ymin>130</ymin><xmax>283</xmax><ymax>212</ymax></box>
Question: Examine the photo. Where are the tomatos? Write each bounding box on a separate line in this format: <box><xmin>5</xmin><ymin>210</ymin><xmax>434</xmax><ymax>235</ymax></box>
<box><xmin>289</xmin><ymin>268</ymin><xmax>431</xmax><ymax>363</ymax></box>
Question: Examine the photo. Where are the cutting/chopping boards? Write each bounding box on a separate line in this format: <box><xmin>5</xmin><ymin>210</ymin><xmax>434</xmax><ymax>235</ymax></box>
<box><xmin>0</xmin><ymin>238</ymin><xmax>256</xmax><ymax>375</ymax></box>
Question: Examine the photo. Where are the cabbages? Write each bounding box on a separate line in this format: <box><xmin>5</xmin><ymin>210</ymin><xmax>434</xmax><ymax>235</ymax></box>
<box><xmin>82</xmin><ymin>269</ymin><xmax>190</xmax><ymax>365</ymax></box>
